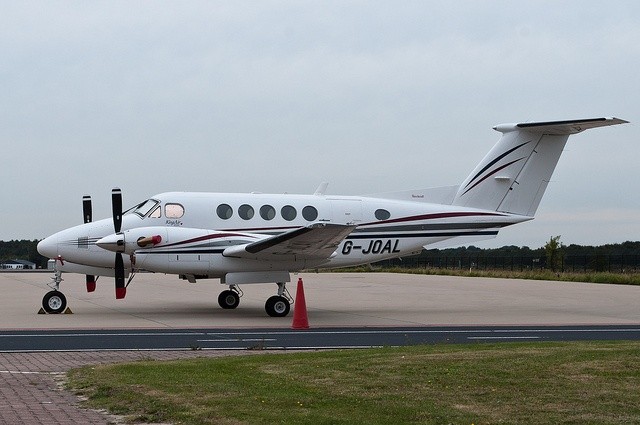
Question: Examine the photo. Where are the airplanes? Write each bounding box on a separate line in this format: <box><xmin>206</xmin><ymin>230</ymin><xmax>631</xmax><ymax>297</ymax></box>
<box><xmin>37</xmin><ymin>116</ymin><xmax>631</xmax><ymax>317</ymax></box>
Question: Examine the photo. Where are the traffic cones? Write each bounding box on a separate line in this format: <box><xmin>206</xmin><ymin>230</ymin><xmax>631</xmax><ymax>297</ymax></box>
<box><xmin>292</xmin><ymin>277</ymin><xmax>309</xmax><ymax>329</ymax></box>
